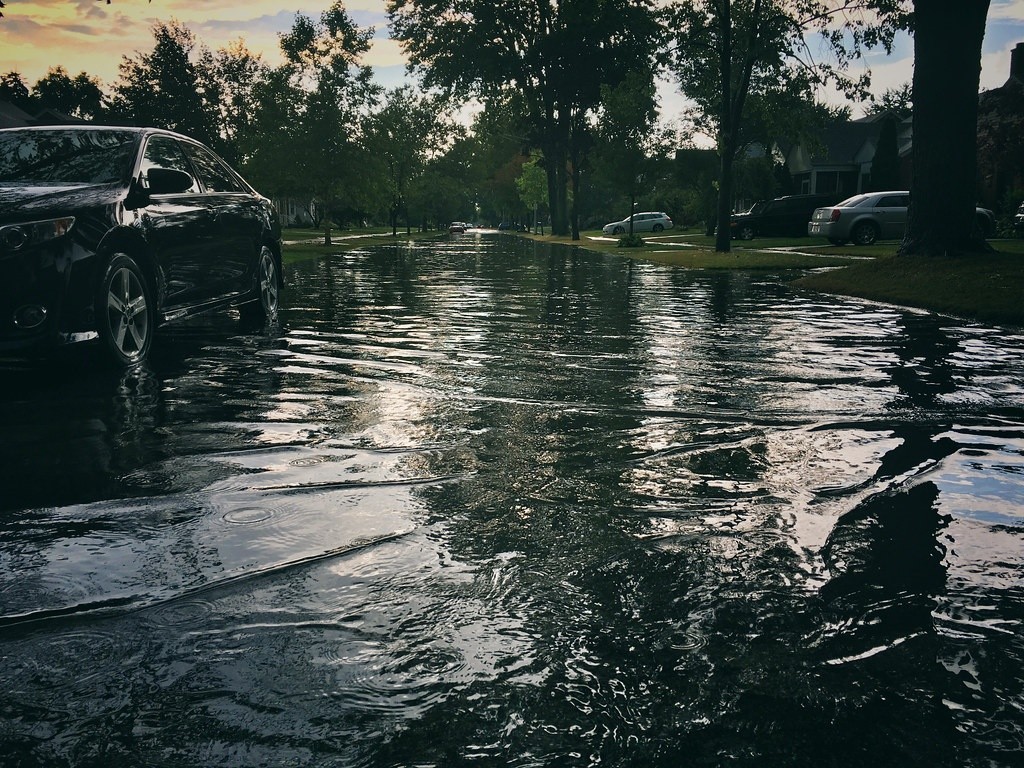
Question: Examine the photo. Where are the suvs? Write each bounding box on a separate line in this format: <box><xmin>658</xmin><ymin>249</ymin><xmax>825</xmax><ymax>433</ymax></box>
<box><xmin>728</xmin><ymin>194</ymin><xmax>831</xmax><ymax>240</ymax></box>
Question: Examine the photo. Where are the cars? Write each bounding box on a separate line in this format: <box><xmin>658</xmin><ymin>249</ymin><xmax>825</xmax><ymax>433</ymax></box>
<box><xmin>498</xmin><ymin>223</ymin><xmax>510</xmax><ymax>230</ymax></box>
<box><xmin>0</xmin><ymin>126</ymin><xmax>285</xmax><ymax>366</ymax></box>
<box><xmin>805</xmin><ymin>191</ymin><xmax>997</xmax><ymax>249</ymax></box>
<box><xmin>603</xmin><ymin>212</ymin><xmax>673</xmax><ymax>236</ymax></box>
<box><xmin>448</xmin><ymin>221</ymin><xmax>473</xmax><ymax>233</ymax></box>
